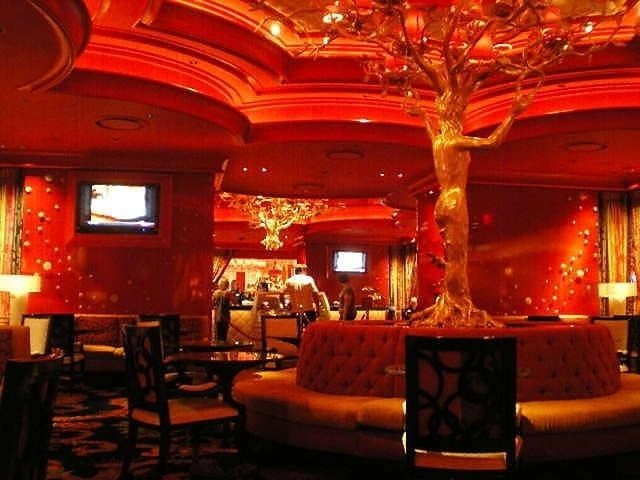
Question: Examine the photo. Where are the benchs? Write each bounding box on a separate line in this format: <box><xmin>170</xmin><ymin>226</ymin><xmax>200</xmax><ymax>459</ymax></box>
<box><xmin>229</xmin><ymin>321</ymin><xmax>637</xmax><ymax>479</ymax></box>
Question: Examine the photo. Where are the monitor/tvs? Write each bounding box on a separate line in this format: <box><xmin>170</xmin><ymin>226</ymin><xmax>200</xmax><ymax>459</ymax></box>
<box><xmin>77</xmin><ymin>182</ymin><xmax>160</xmax><ymax>234</ymax></box>
<box><xmin>333</xmin><ymin>249</ymin><xmax>367</xmax><ymax>274</ymax></box>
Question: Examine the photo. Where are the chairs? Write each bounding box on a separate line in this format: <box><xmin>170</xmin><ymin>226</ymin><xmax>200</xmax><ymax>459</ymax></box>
<box><xmin>399</xmin><ymin>333</ymin><xmax>523</xmax><ymax>480</ymax></box>
<box><xmin>119</xmin><ymin>323</ymin><xmax>239</xmax><ymax>477</ymax></box>
<box><xmin>2</xmin><ymin>309</ymin><xmax>390</xmax><ymax>390</ymax></box>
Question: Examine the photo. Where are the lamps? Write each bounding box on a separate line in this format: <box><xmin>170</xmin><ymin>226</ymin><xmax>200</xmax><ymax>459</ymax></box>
<box><xmin>597</xmin><ymin>279</ymin><xmax>637</xmax><ymax>314</ymax></box>
<box><xmin>242</xmin><ymin>196</ymin><xmax>333</xmax><ymax>252</ymax></box>
<box><xmin>0</xmin><ymin>273</ymin><xmax>42</xmax><ymax>326</ymax></box>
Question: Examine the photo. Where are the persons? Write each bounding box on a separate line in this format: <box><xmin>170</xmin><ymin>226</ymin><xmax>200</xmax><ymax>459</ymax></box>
<box><xmin>212</xmin><ymin>274</ymin><xmax>233</xmax><ymax>343</ymax></box>
<box><xmin>403</xmin><ymin>297</ymin><xmax>423</xmax><ymax>321</ymax></box>
<box><xmin>230</xmin><ymin>278</ymin><xmax>246</xmax><ymax>305</ymax></box>
<box><xmin>277</xmin><ymin>262</ymin><xmax>322</xmax><ymax>325</ymax></box>
<box><xmin>337</xmin><ymin>273</ymin><xmax>357</xmax><ymax>320</ymax></box>
